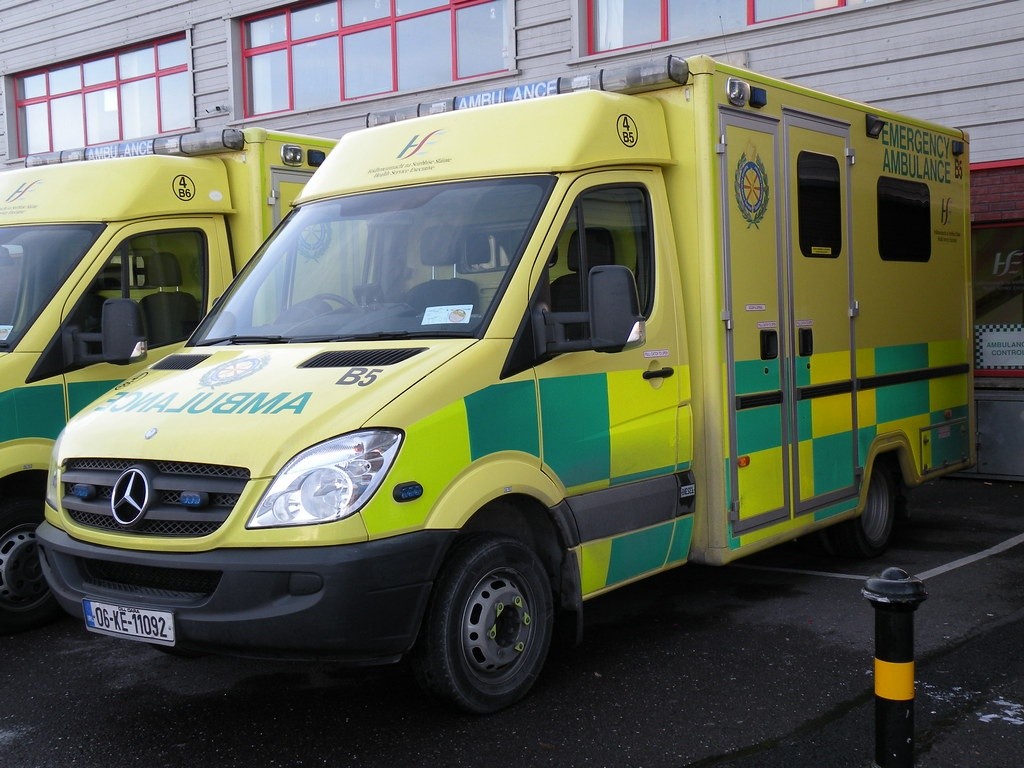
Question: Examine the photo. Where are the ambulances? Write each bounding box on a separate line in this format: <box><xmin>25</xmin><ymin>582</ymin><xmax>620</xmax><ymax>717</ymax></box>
<box><xmin>0</xmin><ymin>128</ymin><xmax>372</xmax><ymax>637</ymax></box>
<box><xmin>32</xmin><ymin>55</ymin><xmax>976</xmax><ymax>719</ymax></box>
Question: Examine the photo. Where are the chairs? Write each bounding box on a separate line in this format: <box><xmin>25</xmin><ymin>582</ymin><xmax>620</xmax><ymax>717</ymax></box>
<box><xmin>138</xmin><ymin>253</ymin><xmax>197</xmax><ymax>346</ymax></box>
<box><xmin>67</xmin><ymin>272</ymin><xmax>109</xmax><ymax>335</ymax></box>
<box><xmin>551</xmin><ymin>227</ymin><xmax>615</xmax><ymax>339</ymax></box>
<box><xmin>403</xmin><ymin>226</ymin><xmax>478</xmax><ymax>316</ymax></box>
